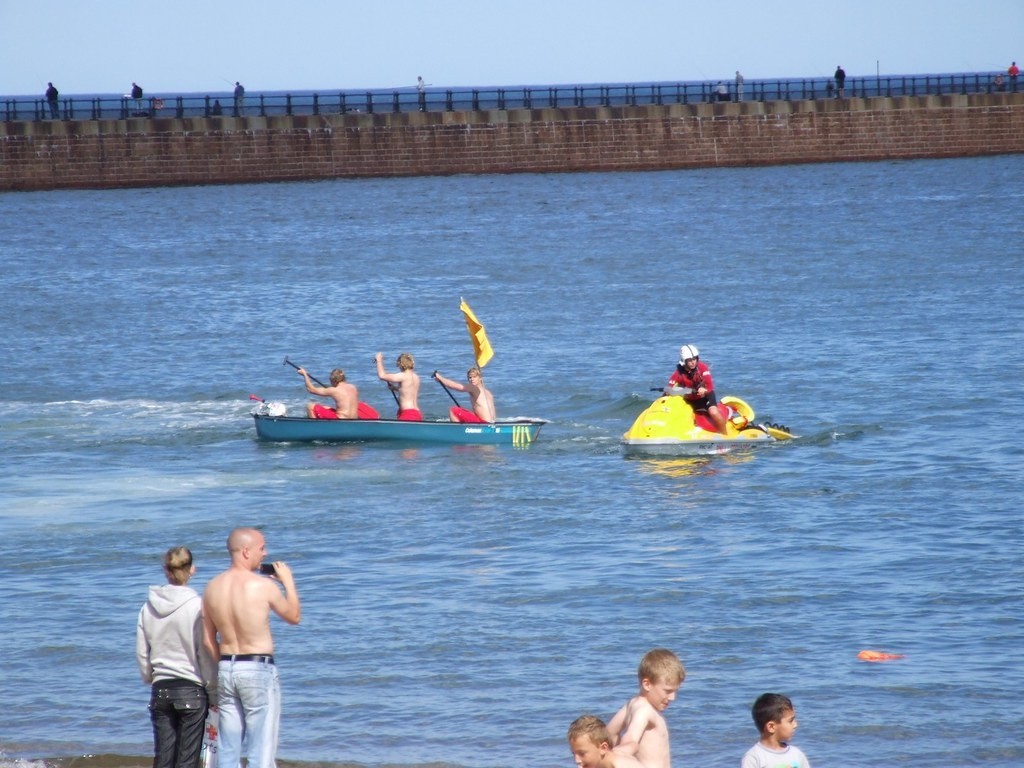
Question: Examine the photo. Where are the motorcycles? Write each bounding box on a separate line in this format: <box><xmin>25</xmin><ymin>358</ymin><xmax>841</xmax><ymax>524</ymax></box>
<box><xmin>619</xmin><ymin>385</ymin><xmax>804</xmax><ymax>455</ymax></box>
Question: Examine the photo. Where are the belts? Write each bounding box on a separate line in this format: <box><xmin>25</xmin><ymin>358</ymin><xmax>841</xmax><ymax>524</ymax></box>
<box><xmin>220</xmin><ymin>654</ymin><xmax>274</xmax><ymax>664</ymax></box>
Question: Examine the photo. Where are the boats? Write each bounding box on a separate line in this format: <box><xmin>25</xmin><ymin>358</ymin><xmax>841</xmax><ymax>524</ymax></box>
<box><xmin>251</xmin><ymin>407</ymin><xmax>546</xmax><ymax>444</ymax></box>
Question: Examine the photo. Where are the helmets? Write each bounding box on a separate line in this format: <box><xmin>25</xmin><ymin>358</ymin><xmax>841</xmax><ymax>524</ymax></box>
<box><xmin>679</xmin><ymin>345</ymin><xmax>699</xmax><ymax>366</ymax></box>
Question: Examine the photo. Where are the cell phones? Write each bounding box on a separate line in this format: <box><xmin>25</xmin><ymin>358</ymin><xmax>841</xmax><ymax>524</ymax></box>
<box><xmin>260</xmin><ymin>563</ymin><xmax>275</xmax><ymax>575</ymax></box>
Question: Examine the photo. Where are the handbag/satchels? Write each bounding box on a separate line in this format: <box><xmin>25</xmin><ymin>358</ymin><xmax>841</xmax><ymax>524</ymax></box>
<box><xmin>198</xmin><ymin>707</ymin><xmax>243</xmax><ymax>768</ymax></box>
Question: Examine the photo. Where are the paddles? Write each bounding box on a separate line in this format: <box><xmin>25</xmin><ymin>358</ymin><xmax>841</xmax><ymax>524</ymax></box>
<box><xmin>373</xmin><ymin>359</ymin><xmax>399</xmax><ymax>405</ymax></box>
<box><xmin>283</xmin><ymin>355</ymin><xmax>327</xmax><ymax>388</ymax></box>
<box><xmin>432</xmin><ymin>370</ymin><xmax>460</xmax><ymax>407</ymax></box>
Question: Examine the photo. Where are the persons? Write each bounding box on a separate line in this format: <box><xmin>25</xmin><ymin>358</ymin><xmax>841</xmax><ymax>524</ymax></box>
<box><xmin>375</xmin><ymin>353</ymin><xmax>423</xmax><ymax>421</ymax></box>
<box><xmin>735</xmin><ymin>71</ymin><xmax>743</xmax><ymax>102</ymax></box>
<box><xmin>1008</xmin><ymin>60</ymin><xmax>1019</xmax><ymax>93</ymax></box>
<box><xmin>298</xmin><ymin>366</ymin><xmax>358</xmax><ymax>419</ymax></box>
<box><xmin>741</xmin><ymin>692</ymin><xmax>811</xmax><ymax>768</ymax></box>
<box><xmin>434</xmin><ymin>367</ymin><xmax>496</xmax><ymax>422</ymax></box>
<box><xmin>714</xmin><ymin>81</ymin><xmax>730</xmax><ymax>101</ymax></box>
<box><xmin>567</xmin><ymin>716</ymin><xmax>647</xmax><ymax>768</ymax></box>
<box><xmin>203</xmin><ymin>527</ymin><xmax>301</xmax><ymax>768</ymax></box>
<box><xmin>233</xmin><ymin>82</ymin><xmax>245</xmax><ymax>116</ymax></box>
<box><xmin>132</xmin><ymin>82</ymin><xmax>143</xmax><ymax>116</ymax></box>
<box><xmin>662</xmin><ymin>344</ymin><xmax>727</xmax><ymax>437</ymax></box>
<box><xmin>136</xmin><ymin>548</ymin><xmax>215</xmax><ymax>768</ymax></box>
<box><xmin>606</xmin><ymin>646</ymin><xmax>687</xmax><ymax>768</ymax></box>
<box><xmin>45</xmin><ymin>82</ymin><xmax>60</xmax><ymax>120</ymax></box>
<box><xmin>834</xmin><ymin>66</ymin><xmax>846</xmax><ymax>97</ymax></box>
<box><xmin>212</xmin><ymin>100</ymin><xmax>222</xmax><ymax>115</ymax></box>
<box><xmin>416</xmin><ymin>76</ymin><xmax>425</xmax><ymax>111</ymax></box>
